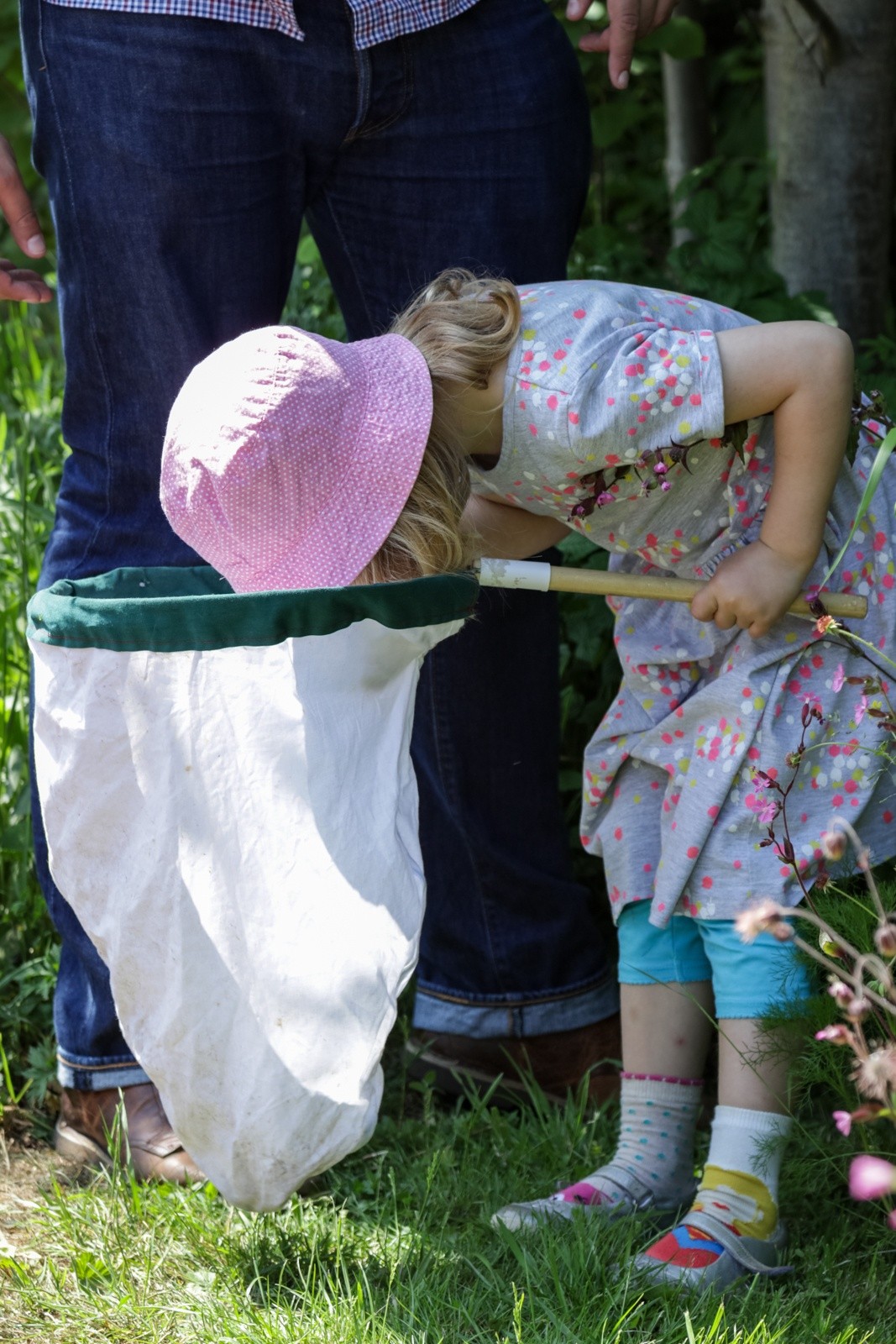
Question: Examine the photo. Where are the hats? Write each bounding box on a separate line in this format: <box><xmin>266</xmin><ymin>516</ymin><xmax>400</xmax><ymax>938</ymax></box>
<box><xmin>159</xmin><ymin>324</ymin><xmax>433</xmax><ymax>595</ymax></box>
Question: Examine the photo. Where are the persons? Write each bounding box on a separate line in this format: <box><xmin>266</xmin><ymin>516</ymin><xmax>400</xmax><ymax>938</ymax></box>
<box><xmin>0</xmin><ymin>0</ymin><xmax>676</xmax><ymax>1187</ymax></box>
<box><xmin>158</xmin><ymin>267</ymin><xmax>896</xmax><ymax>1302</ymax></box>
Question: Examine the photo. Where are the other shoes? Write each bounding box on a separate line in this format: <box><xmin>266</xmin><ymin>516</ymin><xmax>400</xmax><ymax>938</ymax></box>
<box><xmin>608</xmin><ymin>1211</ymin><xmax>796</xmax><ymax>1298</ymax></box>
<box><xmin>490</xmin><ymin>1165</ymin><xmax>698</xmax><ymax>1243</ymax></box>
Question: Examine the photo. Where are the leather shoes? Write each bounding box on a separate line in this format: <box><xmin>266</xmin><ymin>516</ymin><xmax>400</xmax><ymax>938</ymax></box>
<box><xmin>409</xmin><ymin>1010</ymin><xmax>622</xmax><ymax>1119</ymax></box>
<box><xmin>54</xmin><ymin>1082</ymin><xmax>208</xmax><ymax>1187</ymax></box>
